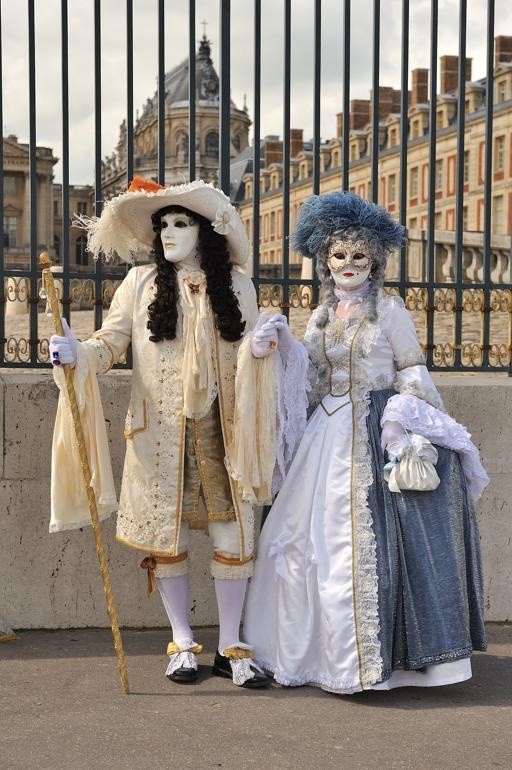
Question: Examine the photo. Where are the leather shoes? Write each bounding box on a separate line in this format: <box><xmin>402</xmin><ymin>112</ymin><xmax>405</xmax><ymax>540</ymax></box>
<box><xmin>212</xmin><ymin>648</ymin><xmax>271</xmax><ymax>690</ymax></box>
<box><xmin>166</xmin><ymin>639</ymin><xmax>203</xmax><ymax>684</ymax></box>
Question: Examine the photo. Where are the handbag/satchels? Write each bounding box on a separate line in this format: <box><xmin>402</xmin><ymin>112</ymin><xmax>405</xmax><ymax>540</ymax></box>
<box><xmin>391</xmin><ymin>431</ymin><xmax>442</xmax><ymax>494</ymax></box>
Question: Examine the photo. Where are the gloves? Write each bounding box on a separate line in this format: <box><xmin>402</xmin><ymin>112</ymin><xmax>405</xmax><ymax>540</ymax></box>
<box><xmin>380</xmin><ymin>421</ymin><xmax>406</xmax><ymax>455</ymax></box>
<box><xmin>48</xmin><ymin>317</ymin><xmax>79</xmax><ymax>367</ymax></box>
<box><xmin>251</xmin><ymin>310</ymin><xmax>299</xmax><ymax>359</ymax></box>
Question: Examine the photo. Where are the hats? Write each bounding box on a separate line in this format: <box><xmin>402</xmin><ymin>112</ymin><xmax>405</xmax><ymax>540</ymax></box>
<box><xmin>70</xmin><ymin>175</ymin><xmax>251</xmax><ymax>268</ymax></box>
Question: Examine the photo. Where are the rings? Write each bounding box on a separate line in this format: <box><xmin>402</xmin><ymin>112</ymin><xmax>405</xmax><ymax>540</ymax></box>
<box><xmin>52</xmin><ymin>351</ymin><xmax>59</xmax><ymax>358</ymax></box>
<box><xmin>269</xmin><ymin>341</ymin><xmax>277</xmax><ymax>350</ymax></box>
<box><xmin>52</xmin><ymin>359</ymin><xmax>61</xmax><ymax>366</ymax></box>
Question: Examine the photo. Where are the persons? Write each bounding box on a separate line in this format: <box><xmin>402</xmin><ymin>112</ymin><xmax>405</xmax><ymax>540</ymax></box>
<box><xmin>48</xmin><ymin>174</ymin><xmax>284</xmax><ymax>689</ymax></box>
<box><xmin>245</xmin><ymin>190</ymin><xmax>488</xmax><ymax>693</ymax></box>
